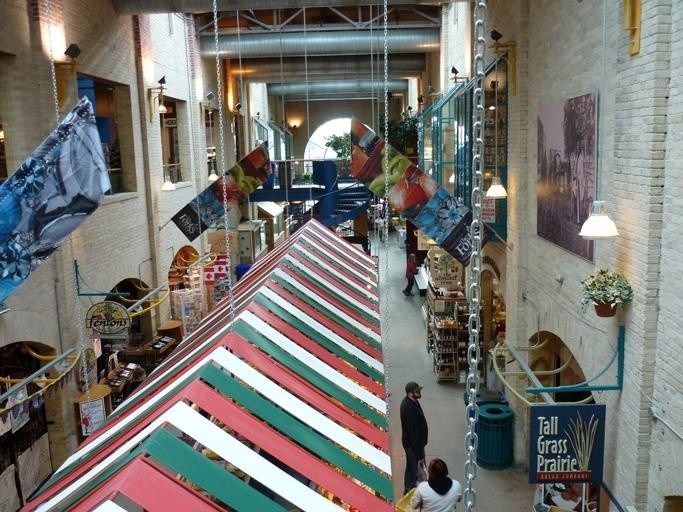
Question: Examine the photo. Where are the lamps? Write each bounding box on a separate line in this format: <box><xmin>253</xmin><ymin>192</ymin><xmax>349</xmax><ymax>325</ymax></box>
<box><xmin>201</xmin><ymin>100</ymin><xmax>218</xmax><ymax>182</ymax></box>
<box><xmin>577</xmin><ymin>199</ymin><xmax>621</xmax><ymax>240</ymax></box>
<box><xmin>162</xmin><ymin>114</ymin><xmax>175</xmax><ymax>192</ymax></box>
<box><xmin>151</xmin><ymin>75</ymin><xmax>168</xmax><ymax>114</ymax></box>
<box><xmin>486</xmin><ymin>29</ymin><xmax>509</xmax><ymax>199</ymax></box>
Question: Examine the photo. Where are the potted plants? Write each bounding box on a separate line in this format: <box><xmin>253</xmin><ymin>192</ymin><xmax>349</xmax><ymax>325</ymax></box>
<box><xmin>577</xmin><ymin>269</ymin><xmax>633</xmax><ymax>315</ymax></box>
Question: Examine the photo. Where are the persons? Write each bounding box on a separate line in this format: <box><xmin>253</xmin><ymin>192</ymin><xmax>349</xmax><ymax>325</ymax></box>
<box><xmin>411</xmin><ymin>460</ymin><xmax>462</xmax><ymax>512</ymax></box>
<box><xmin>399</xmin><ymin>381</ymin><xmax>428</xmax><ymax>495</ymax></box>
<box><xmin>124</xmin><ymin>368</ymin><xmax>147</xmax><ymax>400</ymax></box>
<box><xmin>402</xmin><ymin>254</ymin><xmax>419</xmax><ymax>296</ymax></box>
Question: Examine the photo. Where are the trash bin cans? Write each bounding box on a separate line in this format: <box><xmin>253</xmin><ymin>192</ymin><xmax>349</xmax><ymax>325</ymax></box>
<box><xmin>476</xmin><ymin>404</ymin><xmax>515</xmax><ymax>470</ymax></box>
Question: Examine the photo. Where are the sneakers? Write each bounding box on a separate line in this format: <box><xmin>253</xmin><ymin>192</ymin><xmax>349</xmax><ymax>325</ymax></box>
<box><xmin>403</xmin><ymin>290</ymin><xmax>410</xmax><ymax>296</ymax></box>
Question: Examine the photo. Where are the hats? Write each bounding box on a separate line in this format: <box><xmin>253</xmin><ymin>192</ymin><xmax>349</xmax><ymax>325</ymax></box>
<box><xmin>405</xmin><ymin>382</ymin><xmax>422</xmax><ymax>394</ymax></box>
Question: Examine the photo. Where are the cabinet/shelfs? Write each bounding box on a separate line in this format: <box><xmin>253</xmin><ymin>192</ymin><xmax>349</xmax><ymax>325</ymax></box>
<box><xmin>426</xmin><ymin>281</ymin><xmax>487</xmax><ymax>385</ymax></box>
